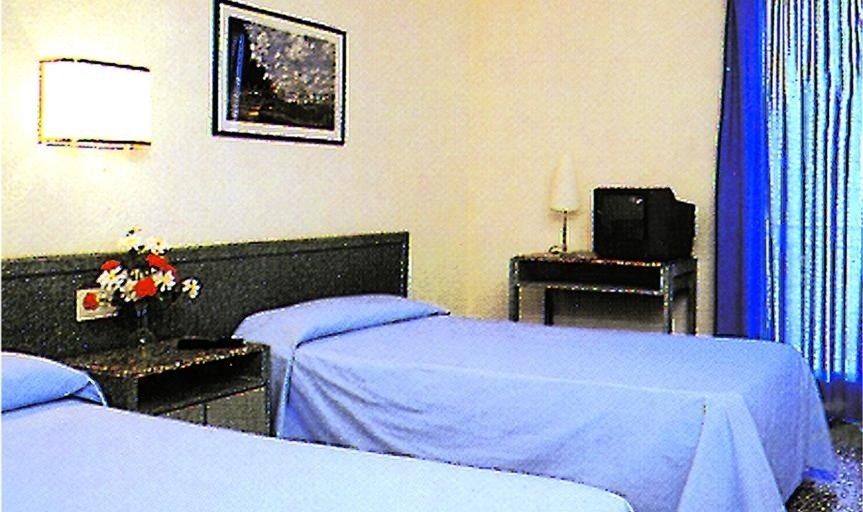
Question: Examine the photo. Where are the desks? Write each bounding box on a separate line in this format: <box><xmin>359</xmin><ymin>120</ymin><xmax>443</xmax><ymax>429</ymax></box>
<box><xmin>507</xmin><ymin>251</ymin><xmax>700</xmax><ymax>336</ymax></box>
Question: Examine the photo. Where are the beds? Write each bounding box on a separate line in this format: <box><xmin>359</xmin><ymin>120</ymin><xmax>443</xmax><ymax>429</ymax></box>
<box><xmin>236</xmin><ymin>294</ymin><xmax>774</xmax><ymax>512</ymax></box>
<box><xmin>1</xmin><ymin>349</ymin><xmax>634</xmax><ymax>512</ymax></box>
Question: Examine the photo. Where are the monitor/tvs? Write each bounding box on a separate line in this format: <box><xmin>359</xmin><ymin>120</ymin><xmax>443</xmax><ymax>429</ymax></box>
<box><xmin>593</xmin><ymin>187</ymin><xmax>697</xmax><ymax>264</ymax></box>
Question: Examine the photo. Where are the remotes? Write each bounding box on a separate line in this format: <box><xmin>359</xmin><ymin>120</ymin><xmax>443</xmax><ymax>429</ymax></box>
<box><xmin>176</xmin><ymin>336</ymin><xmax>245</xmax><ymax>349</ymax></box>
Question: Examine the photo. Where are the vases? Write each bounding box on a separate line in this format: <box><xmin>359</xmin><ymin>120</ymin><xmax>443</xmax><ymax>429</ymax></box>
<box><xmin>123</xmin><ymin>324</ymin><xmax>177</xmax><ymax>364</ymax></box>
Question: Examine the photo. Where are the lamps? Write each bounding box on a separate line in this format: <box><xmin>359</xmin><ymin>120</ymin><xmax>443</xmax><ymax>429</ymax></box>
<box><xmin>551</xmin><ymin>163</ymin><xmax>582</xmax><ymax>255</ymax></box>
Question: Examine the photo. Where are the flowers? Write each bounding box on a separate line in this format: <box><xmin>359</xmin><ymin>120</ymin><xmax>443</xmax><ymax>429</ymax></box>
<box><xmin>89</xmin><ymin>229</ymin><xmax>201</xmax><ymax>327</ymax></box>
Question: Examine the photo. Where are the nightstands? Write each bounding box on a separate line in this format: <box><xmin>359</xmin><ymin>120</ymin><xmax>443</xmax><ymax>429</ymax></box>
<box><xmin>67</xmin><ymin>335</ymin><xmax>284</xmax><ymax>436</ymax></box>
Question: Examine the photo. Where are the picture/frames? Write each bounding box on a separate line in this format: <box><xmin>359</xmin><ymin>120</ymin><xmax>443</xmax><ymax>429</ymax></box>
<box><xmin>210</xmin><ymin>1</ymin><xmax>349</xmax><ymax>146</ymax></box>
